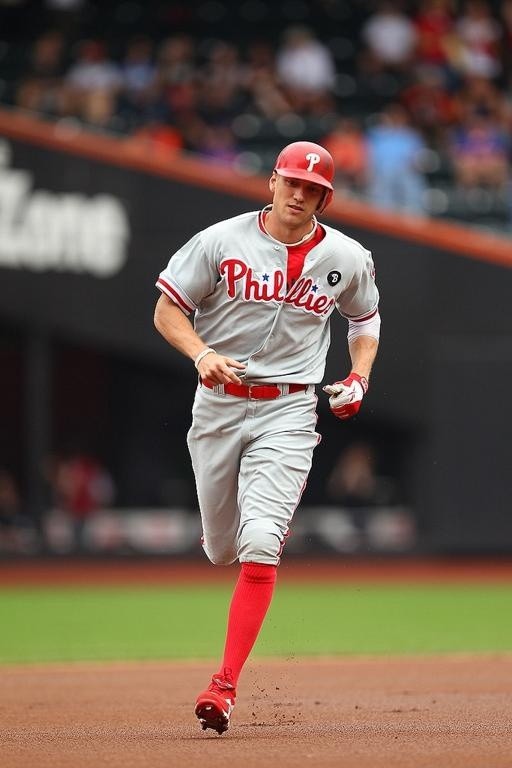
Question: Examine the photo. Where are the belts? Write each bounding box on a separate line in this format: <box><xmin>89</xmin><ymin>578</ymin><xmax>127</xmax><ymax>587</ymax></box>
<box><xmin>200</xmin><ymin>377</ymin><xmax>307</xmax><ymax>400</ymax></box>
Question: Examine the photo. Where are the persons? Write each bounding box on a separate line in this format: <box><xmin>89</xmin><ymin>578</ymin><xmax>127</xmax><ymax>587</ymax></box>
<box><xmin>150</xmin><ymin>141</ymin><xmax>382</xmax><ymax>735</ymax></box>
<box><xmin>0</xmin><ymin>0</ymin><xmax>511</xmax><ymax>237</ymax></box>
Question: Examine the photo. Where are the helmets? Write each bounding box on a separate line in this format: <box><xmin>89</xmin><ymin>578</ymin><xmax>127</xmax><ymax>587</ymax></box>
<box><xmin>274</xmin><ymin>141</ymin><xmax>335</xmax><ymax>215</ymax></box>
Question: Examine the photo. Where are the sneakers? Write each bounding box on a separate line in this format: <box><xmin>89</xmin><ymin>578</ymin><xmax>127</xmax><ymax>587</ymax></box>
<box><xmin>194</xmin><ymin>674</ymin><xmax>237</xmax><ymax>735</ymax></box>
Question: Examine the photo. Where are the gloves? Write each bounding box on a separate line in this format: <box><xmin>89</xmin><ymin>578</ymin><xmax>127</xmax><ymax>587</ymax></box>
<box><xmin>322</xmin><ymin>373</ymin><xmax>368</xmax><ymax>420</ymax></box>
